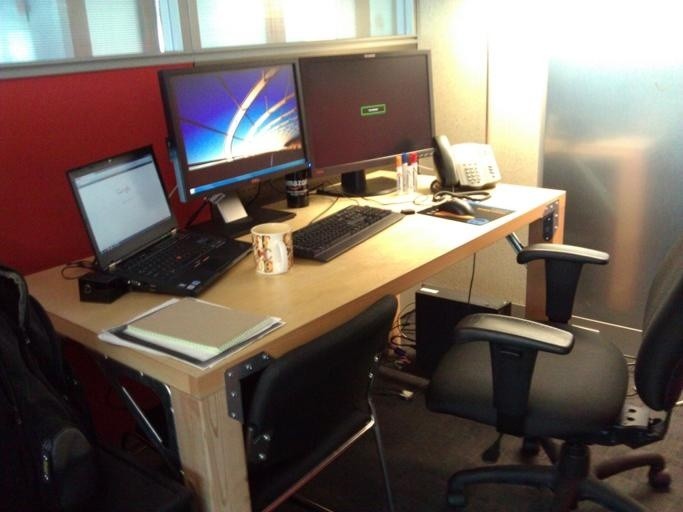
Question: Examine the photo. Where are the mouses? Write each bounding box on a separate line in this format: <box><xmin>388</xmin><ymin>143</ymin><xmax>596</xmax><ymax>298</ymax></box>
<box><xmin>437</xmin><ymin>199</ymin><xmax>475</xmax><ymax>219</ymax></box>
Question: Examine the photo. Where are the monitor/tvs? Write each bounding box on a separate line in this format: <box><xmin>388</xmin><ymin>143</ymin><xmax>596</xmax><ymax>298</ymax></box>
<box><xmin>157</xmin><ymin>55</ymin><xmax>311</xmax><ymax>240</ymax></box>
<box><xmin>299</xmin><ymin>49</ymin><xmax>437</xmax><ymax>197</ymax></box>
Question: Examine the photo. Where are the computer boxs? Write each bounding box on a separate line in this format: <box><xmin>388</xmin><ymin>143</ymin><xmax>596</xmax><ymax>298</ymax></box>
<box><xmin>415</xmin><ymin>283</ymin><xmax>511</xmax><ymax>370</ymax></box>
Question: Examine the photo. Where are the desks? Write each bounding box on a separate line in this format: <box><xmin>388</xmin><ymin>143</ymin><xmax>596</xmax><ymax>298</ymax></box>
<box><xmin>22</xmin><ymin>170</ymin><xmax>568</xmax><ymax>512</ymax></box>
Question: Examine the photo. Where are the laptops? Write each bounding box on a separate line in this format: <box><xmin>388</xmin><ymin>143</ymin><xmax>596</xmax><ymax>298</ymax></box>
<box><xmin>66</xmin><ymin>144</ymin><xmax>253</xmax><ymax>297</ymax></box>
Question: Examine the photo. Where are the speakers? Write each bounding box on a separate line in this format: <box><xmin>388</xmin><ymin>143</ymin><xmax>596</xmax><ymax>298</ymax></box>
<box><xmin>78</xmin><ymin>272</ymin><xmax>128</xmax><ymax>303</ymax></box>
<box><xmin>341</xmin><ymin>169</ymin><xmax>366</xmax><ymax>195</ymax></box>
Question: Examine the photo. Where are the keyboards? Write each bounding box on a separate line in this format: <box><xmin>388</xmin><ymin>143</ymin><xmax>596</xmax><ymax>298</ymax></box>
<box><xmin>292</xmin><ymin>204</ymin><xmax>405</xmax><ymax>262</ymax></box>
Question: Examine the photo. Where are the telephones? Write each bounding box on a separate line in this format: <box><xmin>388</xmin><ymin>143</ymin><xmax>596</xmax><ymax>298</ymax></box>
<box><xmin>433</xmin><ymin>134</ymin><xmax>501</xmax><ymax>189</ymax></box>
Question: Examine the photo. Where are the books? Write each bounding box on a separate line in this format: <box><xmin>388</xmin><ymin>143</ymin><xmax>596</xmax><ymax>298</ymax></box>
<box><xmin>97</xmin><ymin>298</ymin><xmax>287</xmax><ymax>375</ymax></box>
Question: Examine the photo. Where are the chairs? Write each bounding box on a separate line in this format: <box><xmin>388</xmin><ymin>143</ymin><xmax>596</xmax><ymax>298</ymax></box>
<box><xmin>133</xmin><ymin>291</ymin><xmax>397</xmax><ymax>510</ymax></box>
<box><xmin>426</xmin><ymin>240</ymin><xmax>682</xmax><ymax>512</ymax></box>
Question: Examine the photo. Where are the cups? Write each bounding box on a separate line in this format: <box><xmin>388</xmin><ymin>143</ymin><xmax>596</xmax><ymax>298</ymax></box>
<box><xmin>251</xmin><ymin>222</ymin><xmax>293</xmax><ymax>274</ymax></box>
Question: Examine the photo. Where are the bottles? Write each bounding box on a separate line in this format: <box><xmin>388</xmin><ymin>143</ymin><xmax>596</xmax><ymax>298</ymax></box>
<box><xmin>396</xmin><ymin>152</ymin><xmax>418</xmax><ymax>193</ymax></box>
